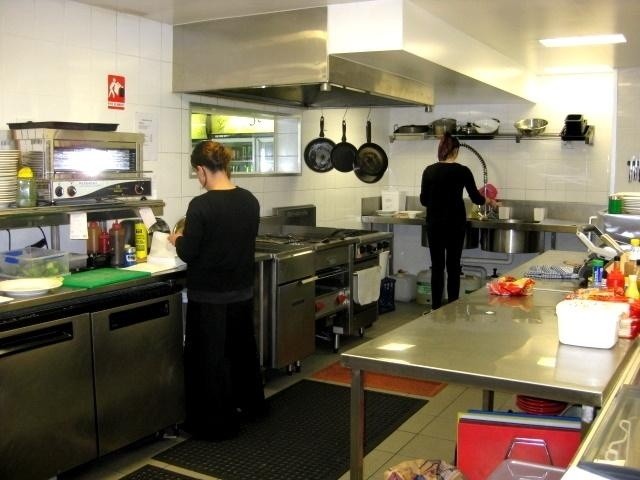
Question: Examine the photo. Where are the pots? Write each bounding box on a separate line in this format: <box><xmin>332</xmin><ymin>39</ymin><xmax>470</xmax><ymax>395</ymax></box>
<box><xmin>304</xmin><ymin>113</ymin><xmax>336</xmax><ymax>172</ymax></box>
<box><xmin>462</xmin><ymin>117</ymin><xmax>500</xmax><ymax>135</ymax></box>
<box><xmin>332</xmin><ymin>119</ymin><xmax>357</xmax><ymax>170</ymax></box>
<box><xmin>353</xmin><ymin>120</ymin><xmax>386</xmax><ymax>184</ymax></box>
<box><xmin>432</xmin><ymin>117</ymin><xmax>459</xmax><ymax>134</ymax></box>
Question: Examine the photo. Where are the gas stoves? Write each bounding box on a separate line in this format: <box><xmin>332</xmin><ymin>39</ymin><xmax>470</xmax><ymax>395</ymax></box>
<box><xmin>329</xmin><ymin>228</ymin><xmax>392</xmax><ymax>258</ymax></box>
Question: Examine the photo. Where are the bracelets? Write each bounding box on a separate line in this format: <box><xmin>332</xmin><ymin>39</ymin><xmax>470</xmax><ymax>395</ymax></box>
<box><xmin>487</xmin><ymin>199</ymin><xmax>493</xmax><ymax>206</ymax></box>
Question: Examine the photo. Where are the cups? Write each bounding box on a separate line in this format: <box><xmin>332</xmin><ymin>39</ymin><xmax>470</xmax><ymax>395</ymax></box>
<box><xmin>607</xmin><ymin>198</ymin><xmax>623</xmax><ymax>214</ymax></box>
<box><xmin>407</xmin><ymin>213</ymin><xmax>417</xmax><ymax>219</ymax></box>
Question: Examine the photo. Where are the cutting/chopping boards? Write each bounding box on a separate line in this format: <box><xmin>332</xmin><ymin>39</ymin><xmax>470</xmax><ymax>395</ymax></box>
<box><xmin>62</xmin><ymin>267</ymin><xmax>150</xmax><ymax>290</ymax></box>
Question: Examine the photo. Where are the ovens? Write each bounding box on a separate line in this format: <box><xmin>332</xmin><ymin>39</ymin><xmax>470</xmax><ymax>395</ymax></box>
<box><xmin>12</xmin><ymin>128</ymin><xmax>153</xmax><ymax>201</ymax></box>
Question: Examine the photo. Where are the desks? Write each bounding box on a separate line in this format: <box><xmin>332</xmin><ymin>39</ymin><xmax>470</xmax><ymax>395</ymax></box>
<box><xmin>341</xmin><ymin>247</ymin><xmax>640</xmax><ymax>480</ymax></box>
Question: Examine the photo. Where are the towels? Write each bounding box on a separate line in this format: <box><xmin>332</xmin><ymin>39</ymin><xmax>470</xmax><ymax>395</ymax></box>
<box><xmin>379</xmin><ymin>250</ymin><xmax>391</xmax><ymax>280</ymax></box>
<box><xmin>353</xmin><ymin>265</ymin><xmax>381</xmax><ymax>306</ymax></box>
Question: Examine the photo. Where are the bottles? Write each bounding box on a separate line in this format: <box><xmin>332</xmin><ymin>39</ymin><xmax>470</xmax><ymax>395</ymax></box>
<box><xmin>18</xmin><ymin>165</ymin><xmax>37</xmax><ymax>208</ymax></box>
<box><xmin>109</xmin><ymin>222</ymin><xmax>125</xmax><ymax>266</ymax></box>
<box><xmin>589</xmin><ymin>238</ymin><xmax>640</xmax><ymax>300</ymax></box>
<box><xmin>223</xmin><ymin>145</ymin><xmax>252</xmax><ymax>160</ymax></box>
<box><xmin>87</xmin><ymin>222</ymin><xmax>101</xmax><ymax>258</ymax></box>
<box><xmin>100</xmin><ymin>232</ymin><xmax>110</xmax><ymax>253</ymax></box>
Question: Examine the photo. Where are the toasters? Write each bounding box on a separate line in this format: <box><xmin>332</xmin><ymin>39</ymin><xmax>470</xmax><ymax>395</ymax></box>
<box><xmin>122</xmin><ymin>217</ymin><xmax>172</xmax><ymax>253</ymax></box>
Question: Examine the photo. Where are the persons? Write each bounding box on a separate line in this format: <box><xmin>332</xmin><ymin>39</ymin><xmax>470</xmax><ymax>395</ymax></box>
<box><xmin>167</xmin><ymin>136</ymin><xmax>261</xmax><ymax>444</ymax></box>
<box><xmin>419</xmin><ymin>133</ymin><xmax>499</xmax><ymax>312</ymax></box>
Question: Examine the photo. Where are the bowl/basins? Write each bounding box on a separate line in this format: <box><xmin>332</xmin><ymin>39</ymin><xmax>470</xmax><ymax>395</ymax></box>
<box><xmin>173</xmin><ymin>217</ymin><xmax>186</xmax><ymax>238</ymax></box>
<box><xmin>513</xmin><ymin>117</ymin><xmax>548</xmax><ymax>136</ymax></box>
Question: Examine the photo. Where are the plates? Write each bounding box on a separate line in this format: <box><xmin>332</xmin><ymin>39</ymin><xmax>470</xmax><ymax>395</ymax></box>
<box><xmin>614</xmin><ymin>192</ymin><xmax>640</xmax><ymax>216</ymax></box>
<box><xmin>515</xmin><ymin>395</ymin><xmax>565</xmax><ymax>418</ymax></box>
<box><xmin>0</xmin><ymin>279</ymin><xmax>63</xmax><ymax>298</ymax></box>
<box><xmin>376</xmin><ymin>209</ymin><xmax>424</xmax><ymax>217</ymax></box>
<box><xmin>0</xmin><ymin>149</ymin><xmax>22</xmax><ymax>210</ymax></box>
<box><xmin>25</xmin><ymin>150</ymin><xmax>46</xmax><ymax>198</ymax></box>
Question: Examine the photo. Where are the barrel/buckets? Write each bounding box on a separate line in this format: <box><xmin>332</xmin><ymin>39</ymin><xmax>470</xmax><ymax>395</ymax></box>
<box><xmin>415</xmin><ymin>266</ymin><xmax>432</xmax><ymax>305</ymax></box>
<box><xmin>458</xmin><ymin>272</ymin><xmax>481</xmax><ymax>298</ymax></box>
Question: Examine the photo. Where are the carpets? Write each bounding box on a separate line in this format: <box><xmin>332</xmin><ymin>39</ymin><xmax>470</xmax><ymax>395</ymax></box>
<box><xmin>118</xmin><ymin>463</ymin><xmax>202</xmax><ymax>480</ymax></box>
<box><xmin>308</xmin><ymin>356</ymin><xmax>447</xmax><ymax>398</ymax></box>
<box><xmin>151</xmin><ymin>378</ymin><xmax>428</xmax><ymax>480</ymax></box>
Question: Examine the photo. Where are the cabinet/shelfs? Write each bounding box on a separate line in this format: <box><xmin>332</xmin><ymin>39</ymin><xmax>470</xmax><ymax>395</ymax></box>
<box><xmin>316</xmin><ymin>243</ymin><xmax>349</xmax><ymax>270</ymax></box>
<box><xmin>256</xmin><ymin>243</ymin><xmax>316</xmax><ymax>369</ymax></box>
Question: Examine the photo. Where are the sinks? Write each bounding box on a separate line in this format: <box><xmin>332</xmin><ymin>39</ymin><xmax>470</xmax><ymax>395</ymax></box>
<box><xmin>480</xmin><ymin>217</ymin><xmax>544</xmax><ymax>253</ymax></box>
<box><xmin>421</xmin><ymin>216</ymin><xmax>479</xmax><ymax>248</ymax></box>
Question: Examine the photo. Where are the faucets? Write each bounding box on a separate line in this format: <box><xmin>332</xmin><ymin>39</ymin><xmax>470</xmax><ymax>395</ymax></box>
<box><xmin>477</xmin><ymin>205</ymin><xmax>482</xmax><ymax>212</ymax></box>
